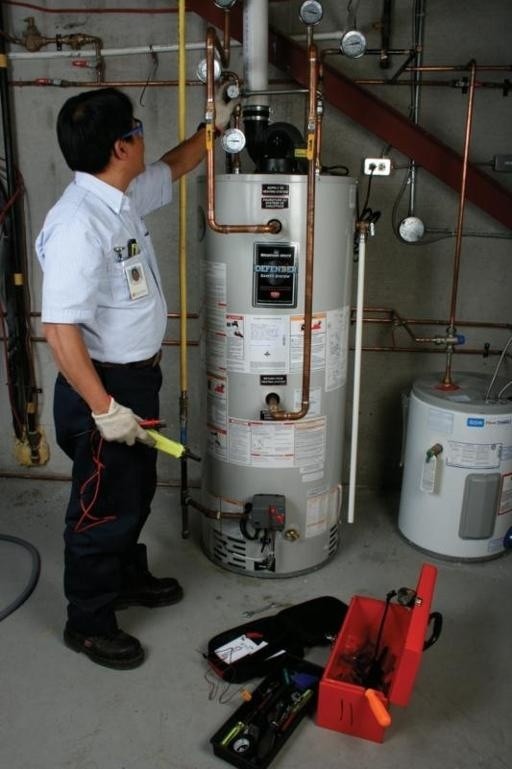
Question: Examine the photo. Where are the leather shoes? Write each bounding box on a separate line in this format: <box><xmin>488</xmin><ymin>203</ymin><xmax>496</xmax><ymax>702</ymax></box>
<box><xmin>64</xmin><ymin>567</ymin><xmax>144</xmax><ymax>670</ymax></box>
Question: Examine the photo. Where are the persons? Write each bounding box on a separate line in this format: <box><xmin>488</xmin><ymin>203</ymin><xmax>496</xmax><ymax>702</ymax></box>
<box><xmin>33</xmin><ymin>80</ymin><xmax>242</xmax><ymax>670</ymax></box>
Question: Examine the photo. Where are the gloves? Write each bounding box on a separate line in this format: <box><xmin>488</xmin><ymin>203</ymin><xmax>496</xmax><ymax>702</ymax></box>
<box><xmin>90</xmin><ymin>395</ymin><xmax>150</xmax><ymax>446</ymax></box>
<box><xmin>198</xmin><ymin>82</ymin><xmax>241</xmax><ymax>138</ymax></box>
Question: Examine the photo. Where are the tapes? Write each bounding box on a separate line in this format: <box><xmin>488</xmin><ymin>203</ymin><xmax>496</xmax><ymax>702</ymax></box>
<box><xmin>228</xmin><ymin>735</ymin><xmax>256</xmax><ymax>757</ymax></box>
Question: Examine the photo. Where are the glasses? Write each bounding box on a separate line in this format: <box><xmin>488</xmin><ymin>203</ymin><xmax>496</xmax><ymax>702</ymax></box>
<box><xmin>122</xmin><ymin>117</ymin><xmax>144</xmax><ymax>139</ymax></box>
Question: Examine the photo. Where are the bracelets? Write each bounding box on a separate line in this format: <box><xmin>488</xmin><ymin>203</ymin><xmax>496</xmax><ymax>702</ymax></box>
<box><xmin>197</xmin><ymin>123</ymin><xmax>221</xmax><ymax>137</ymax></box>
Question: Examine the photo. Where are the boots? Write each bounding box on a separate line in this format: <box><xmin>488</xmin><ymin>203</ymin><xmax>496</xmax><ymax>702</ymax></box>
<box><xmin>115</xmin><ymin>543</ymin><xmax>183</xmax><ymax>611</ymax></box>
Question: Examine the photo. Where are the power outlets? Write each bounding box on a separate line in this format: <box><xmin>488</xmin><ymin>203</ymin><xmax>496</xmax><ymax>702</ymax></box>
<box><xmin>363</xmin><ymin>156</ymin><xmax>393</xmax><ymax>177</ymax></box>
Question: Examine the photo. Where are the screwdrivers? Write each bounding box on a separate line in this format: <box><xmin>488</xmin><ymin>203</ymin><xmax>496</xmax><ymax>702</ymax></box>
<box><xmin>221</xmin><ymin>691</ymin><xmax>271</xmax><ymax>747</ymax></box>
<box><xmin>360</xmin><ymin>675</ymin><xmax>391</xmax><ymax>728</ymax></box>
<box><xmin>257</xmin><ymin>708</ymin><xmax>282</xmax><ymax>759</ymax></box>
